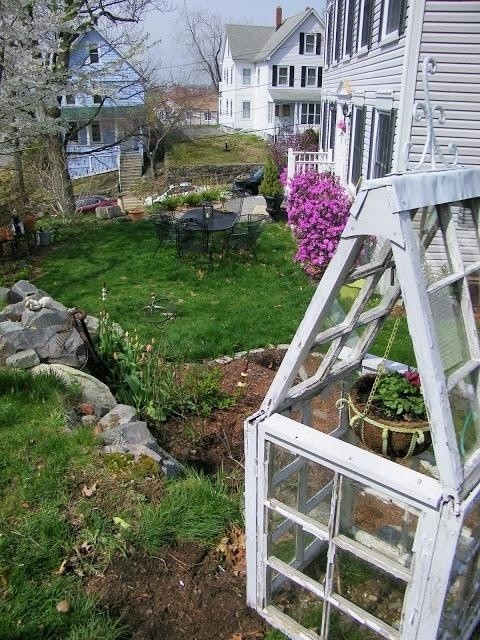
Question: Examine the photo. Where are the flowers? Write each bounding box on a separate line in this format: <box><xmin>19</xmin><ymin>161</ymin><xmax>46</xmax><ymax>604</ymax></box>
<box><xmin>376</xmin><ymin>366</ymin><xmax>424</xmax><ymax>417</ymax></box>
<box><xmin>337</xmin><ymin>119</ymin><xmax>346</xmax><ymax>129</ymax></box>
<box><xmin>23</xmin><ymin>205</ymin><xmax>33</xmax><ymax>217</ymax></box>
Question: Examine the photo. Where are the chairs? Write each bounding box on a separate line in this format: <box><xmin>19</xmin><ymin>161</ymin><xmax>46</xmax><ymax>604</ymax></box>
<box><xmin>149</xmin><ymin>190</ymin><xmax>269</xmax><ymax>263</ymax></box>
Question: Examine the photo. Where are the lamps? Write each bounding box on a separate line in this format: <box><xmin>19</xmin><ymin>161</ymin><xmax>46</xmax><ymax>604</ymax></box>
<box><xmin>330</xmin><ymin>101</ymin><xmax>336</xmax><ymax>113</ymax></box>
<box><xmin>341</xmin><ymin>102</ymin><xmax>351</xmax><ymax>119</ymax></box>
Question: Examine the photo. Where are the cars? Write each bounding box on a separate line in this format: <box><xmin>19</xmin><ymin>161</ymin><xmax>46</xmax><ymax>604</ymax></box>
<box><xmin>145</xmin><ymin>182</ymin><xmax>209</xmax><ymax>207</ymax></box>
<box><xmin>75</xmin><ymin>195</ymin><xmax>117</xmax><ymax>213</ymax></box>
<box><xmin>234</xmin><ymin>168</ymin><xmax>264</xmax><ymax>196</ymax></box>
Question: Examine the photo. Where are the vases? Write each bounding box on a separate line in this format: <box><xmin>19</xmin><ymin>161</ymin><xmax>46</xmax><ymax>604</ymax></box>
<box><xmin>128</xmin><ymin>210</ymin><xmax>144</xmax><ymax>221</ymax></box>
<box><xmin>24</xmin><ymin>218</ymin><xmax>36</xmax><ymax>231</ymax></box>
